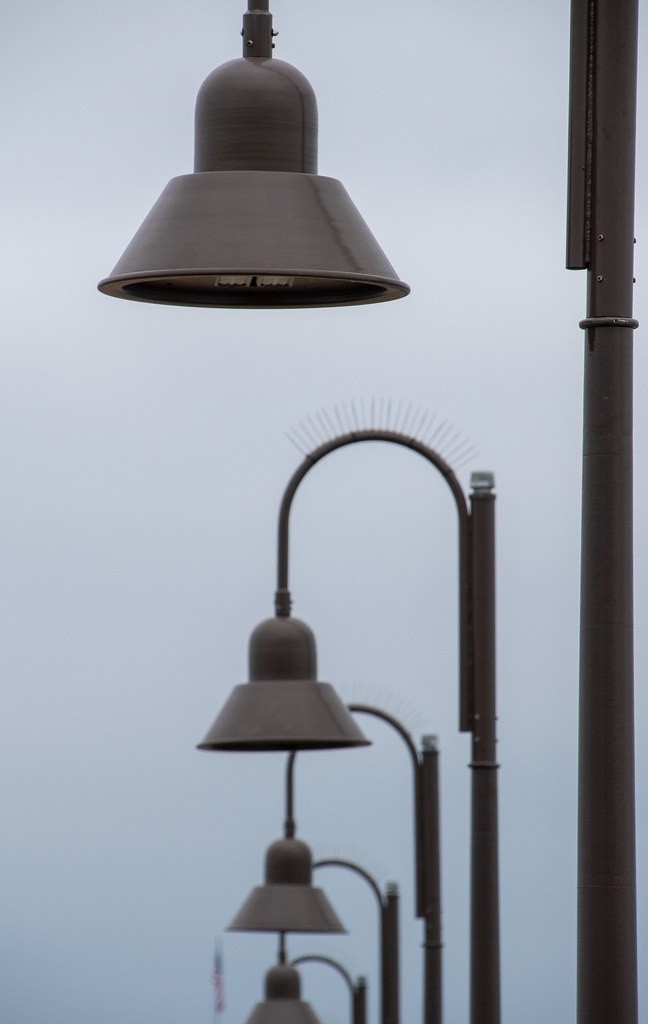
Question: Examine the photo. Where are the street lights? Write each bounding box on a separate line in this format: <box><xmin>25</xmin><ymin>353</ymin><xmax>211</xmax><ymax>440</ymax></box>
<box><xmin>242</xmin><ymin>857</ymin><xmax>400</xmax><ymax>1024</ymax></box>
<box><xmin>95</xmin><ymin>0</ymin><xmax>641</xmax><ymax>1024</ymax></box>
<box><xmin>197</xmin><ymin>428</ymin><xmax>505</xmax><ymax>1024</ymax></box>
<box><xmin>225</xmin><ymin>704</ymin><xmax>444</xmax><ymax>1024</ymax></box>
